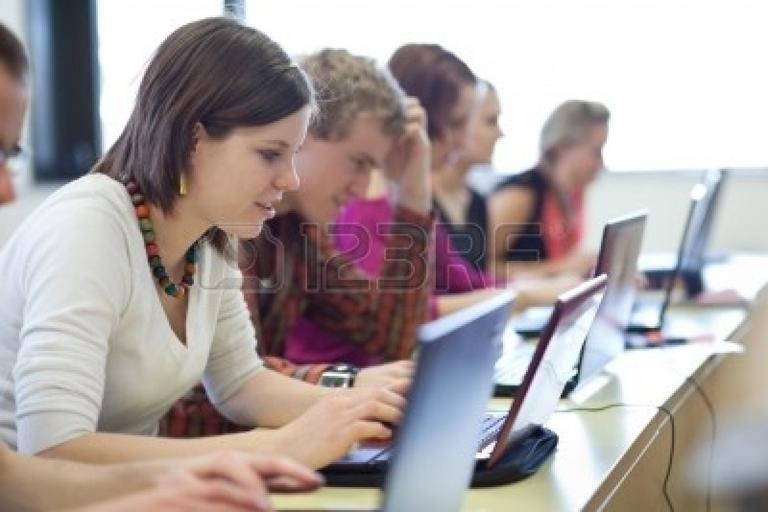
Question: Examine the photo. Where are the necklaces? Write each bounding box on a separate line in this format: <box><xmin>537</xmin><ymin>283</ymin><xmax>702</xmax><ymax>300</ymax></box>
<box><xmin>109</xmin><ymin>165</ymin><xmax>207</xmax><ymax>302</ymax></box>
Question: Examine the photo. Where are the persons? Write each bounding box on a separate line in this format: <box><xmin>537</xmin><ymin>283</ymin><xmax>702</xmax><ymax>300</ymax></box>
<box><xmin>155</xmin><ymin>46</ymin><xmax>437</xmax><ymax>438</ymax></box>
<box><xmin>428</xmin><ymin>78</ymin><xmax>585</xmax><ymax>307</ymax></box>
<box><xmin>480</xmin><ymin>99</ymin><xmax>648</xmax><ymax>287</ymax></box>
<box><xmin>282</xmin><ymin>38</ymin><xmax>547</xmax><ymax>362</ymax></box>
<box><xmin>4</xmin><ymin>18</ymin><xmax>405</xmax><ymax>476</ymax></box>
<box><xmin>0</xmin><ymin>24</ymin><xmax>326</xmax><ymax>510</ymax></box>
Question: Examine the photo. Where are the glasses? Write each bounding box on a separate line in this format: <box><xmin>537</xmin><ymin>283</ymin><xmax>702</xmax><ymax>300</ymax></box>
<box><xmin>0</xmin><ymin>144</ymin><xmax>31</xmax><ymax>176</ymax></box>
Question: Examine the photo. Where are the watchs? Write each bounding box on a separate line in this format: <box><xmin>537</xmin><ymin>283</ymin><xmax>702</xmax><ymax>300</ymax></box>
<box><xmin>319</xmin><ymin>362</ymin><xmax>362</xmax><ymax>389</ymax></box>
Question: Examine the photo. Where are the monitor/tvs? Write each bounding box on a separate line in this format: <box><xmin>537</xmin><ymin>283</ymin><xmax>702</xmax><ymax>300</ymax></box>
<box><xmin>379</xmin><ymin>294</ymin><xmax>514</xmax><ymax>507</ymax></box>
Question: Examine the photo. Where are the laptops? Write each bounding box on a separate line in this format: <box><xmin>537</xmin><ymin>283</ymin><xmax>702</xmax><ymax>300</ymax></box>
<box><xmin>492</xmin><ymin>211</ymin><xmax>649</xmax><ymax>399</ymax></box>
<box><xmin>511</xmin><ymin>191</ymin><xmax>702</xmax><ymax>338</ymax></box>
<box><xmin>316</xmin><ymin>274</ymin><xmax>611</xmax><ymax>489</ymax></box>
<box><xmin>641</xmin><ymin>173</ymin><xmax>724</xmax><ymax>297</ymax></box>
